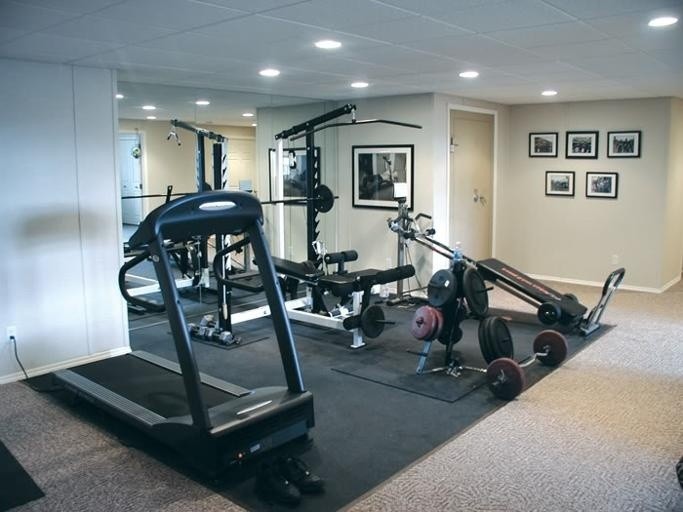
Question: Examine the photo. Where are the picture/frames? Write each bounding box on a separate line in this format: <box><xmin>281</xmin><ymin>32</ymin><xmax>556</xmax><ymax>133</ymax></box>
<box><xmin>352</xmin><ymin>143</ymin><xmax>414</xmax><ymax>210</ymax></box>
<box><xmin>545</xmin><ymin>171</ymin><xmax>575</xmax><ymax>196</ymax></box>
<box><xmin>586</xmin><ymin>172</ymin><xmax>618</xmax><ymax>198</ymax></box>
<box><xmin>529</xmin><ymin>133</ymin><xmax>558</xmax><ymax>157</ymax></box>
<box><xmin>566</xmin><ymin>131</ymin><xmax>598</xmax><ymax>159</ymax></box>
<box><xmin>268</xmin><ymin>147</ymin><xmax>320</xmax><ymax>205</ymax></box>
<box><xmin>607</xmin><ymin>131</ymin><xmax>641</xmax><ymax>158</ymax></box>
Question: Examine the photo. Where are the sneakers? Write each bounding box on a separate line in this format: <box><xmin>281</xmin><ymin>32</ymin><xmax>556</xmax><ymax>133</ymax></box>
<box><xmin>255</xmin><ymin>455</ymin><xmax>322</xmax><ymax>506</ymax></box>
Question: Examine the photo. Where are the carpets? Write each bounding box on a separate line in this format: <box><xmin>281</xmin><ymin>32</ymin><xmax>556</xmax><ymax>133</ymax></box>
<box><xmin>18</xmin><ymin>291</ymin><xmax>619</xmax><ymax>512</ymax></box>
<box><xmin>0</xmin><ymin>441</ymin><xmax>45</xmax><ymax>512</ymax></box>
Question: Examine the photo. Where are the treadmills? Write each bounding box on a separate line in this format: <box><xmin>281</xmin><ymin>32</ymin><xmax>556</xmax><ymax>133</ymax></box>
<box><xmin>52</xmin><ymin>189</ymin><xmax>315</xmax><ymax>466</ymax></box>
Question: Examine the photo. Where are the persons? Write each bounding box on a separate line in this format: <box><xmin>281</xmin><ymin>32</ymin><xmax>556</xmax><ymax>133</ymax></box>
<box><xmin>286</xmin><ymin>151</ymin><xmax>296</xmax><ymax>173</ymax></box>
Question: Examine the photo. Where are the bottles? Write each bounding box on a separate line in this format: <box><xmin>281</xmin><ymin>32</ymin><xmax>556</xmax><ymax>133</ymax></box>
<box><xmin>453</xmin><ymin>241</ymin><xmax>464</xmax><ymax>261</ymax></box>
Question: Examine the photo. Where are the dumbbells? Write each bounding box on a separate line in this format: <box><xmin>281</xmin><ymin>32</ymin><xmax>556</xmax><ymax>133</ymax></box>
<box><xmin>185</xmin><ymin>313</ymin><xmax>232</xmax><ymax>344</ymax></box>
<box><xmin>485</xmin><ymin>330</ymin><xmax>568</xmax><ymax>401</ymax></box>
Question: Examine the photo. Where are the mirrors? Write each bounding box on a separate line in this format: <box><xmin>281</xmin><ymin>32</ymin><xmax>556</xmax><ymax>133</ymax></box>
<box><xmin>115</xmin><ymin>80</ymin><xmax>337</xmax><ymax>332</ymax></box>
